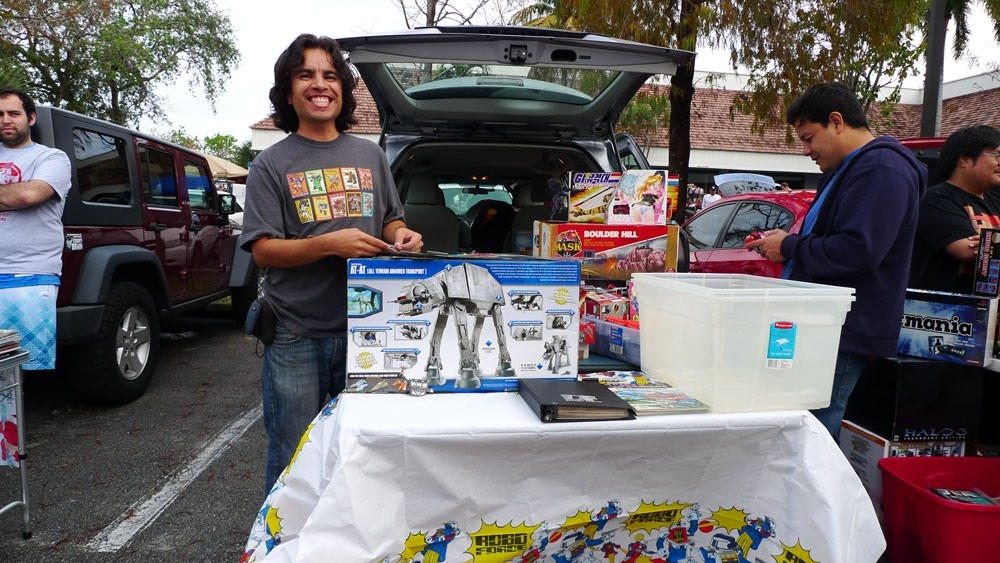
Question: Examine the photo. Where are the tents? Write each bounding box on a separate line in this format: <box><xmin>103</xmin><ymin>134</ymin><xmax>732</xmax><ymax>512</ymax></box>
<box><xmin>186</xmin><ymin>151</ymin><xmax>250</xmax><ymax>180</ymax></box>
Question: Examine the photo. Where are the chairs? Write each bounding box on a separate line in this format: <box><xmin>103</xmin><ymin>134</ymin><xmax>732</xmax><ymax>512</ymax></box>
<box><xmin>401</xmin><ymin>171</ymin><xmax>460</xmax><ymax>252</ymax></box>
<box><xmin>503</xmin><ymin>170</ymin><xmax>569</xmax><ymax>254</ymax></box>
<box><xmin>511</xmin><ymin>183</ymin><xmax>536</xmax><ymax>208</ymax></box>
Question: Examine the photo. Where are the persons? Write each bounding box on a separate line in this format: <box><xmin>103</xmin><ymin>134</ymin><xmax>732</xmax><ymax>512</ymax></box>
<box><xmin>781</xmin><ymin>182</ymin><xmax>790</xmax><ymax>190</ymax></box>
<box><xmin>0</xmin><ymin>90</ymin><xmax>73</xmax><ymax>370</ymax></box>
<box><xmin>701</xmin><ymin>184</ymin><xmax>721</xmax><ymax>210</ymax></box>
<box><xmin>240</xmin><ymin>33</ymin><xmax>424</xmax><ymax>503</ymax></box>
<box><xmin>746</xmin><ymin>83</ymin><xmax>927</xmax><ymax>433</ymax></box>
<box><xmin>912</xmin><ymin>125</ymin><xmax>1000</xmax><ymax>300</ymax></box>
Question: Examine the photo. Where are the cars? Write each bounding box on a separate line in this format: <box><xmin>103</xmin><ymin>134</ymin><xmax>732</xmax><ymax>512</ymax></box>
<box><xmin>680</xmin><ymin>139</ymin><xmax>948</xmax><ymax>283</ymax></box>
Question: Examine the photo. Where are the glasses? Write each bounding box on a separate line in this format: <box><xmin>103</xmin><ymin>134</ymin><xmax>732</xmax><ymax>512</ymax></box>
<box><xmin>980</xmin><ymin>153</ymin><xmax>1000</xmax><ymax>162</ymax></box>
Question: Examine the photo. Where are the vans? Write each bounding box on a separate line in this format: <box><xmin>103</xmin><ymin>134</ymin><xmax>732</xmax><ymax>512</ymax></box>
<box><xmin>332</xmin><ymin>26</ymin><xmax>698</xmax><ymax>276</ymax></box>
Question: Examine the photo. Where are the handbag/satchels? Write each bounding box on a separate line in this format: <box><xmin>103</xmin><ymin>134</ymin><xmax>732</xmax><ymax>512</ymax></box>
<box><xmin>244</xmin><ymin>297</ymin><xmax>277</xmax><ymax>347</ymax></box>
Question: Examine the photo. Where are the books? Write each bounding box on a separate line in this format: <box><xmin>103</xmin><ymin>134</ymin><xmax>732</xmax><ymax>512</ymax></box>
<box><xmin>930</xmin><ymin>488</ymin><xmax>993</xmax><ymax>505</ymax></box>
<box><xmin>519</xmin><ymin>378</ymin><xmax>638</xmax><ymax>423</ymax></box>
<box><xmin>578</xmin><ymin>371</ymin><xmax>710</xmax><ymax>416</ymax></box>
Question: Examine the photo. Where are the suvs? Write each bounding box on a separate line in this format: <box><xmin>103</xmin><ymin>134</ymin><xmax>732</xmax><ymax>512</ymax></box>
<box><xmin>31</xmin><ymin>104</ymin><xmax>261</xmax><ymax>407</ymax></box>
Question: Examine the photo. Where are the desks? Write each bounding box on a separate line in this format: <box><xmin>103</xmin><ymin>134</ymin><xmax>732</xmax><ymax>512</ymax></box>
<box><xmin>238</xmin><ymin>384</ymin><xmax>888</xmax><ymax>563</ymax></box>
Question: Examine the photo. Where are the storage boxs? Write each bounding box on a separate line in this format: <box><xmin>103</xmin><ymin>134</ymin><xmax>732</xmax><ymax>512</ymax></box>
<box><xmin>875</xmin><ymin>456</ymin><xmax>1000</xmax><ymax>563</ymax></box>
<box><xmin>897</xmin><ymin>228</ymin><xmax>1000</xmax><ymax>372</ymax></box>
<box><xmin>344</xmin><ymin>170</ymin><xmax>857</xmax><ymax>414</ymax></box>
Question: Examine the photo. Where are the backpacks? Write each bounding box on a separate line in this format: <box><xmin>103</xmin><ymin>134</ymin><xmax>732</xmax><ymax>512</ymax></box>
<box><xmin>471</xmin><ymin>201</ymin><xmax>516</xmax><ymax>254</ymax></box>
